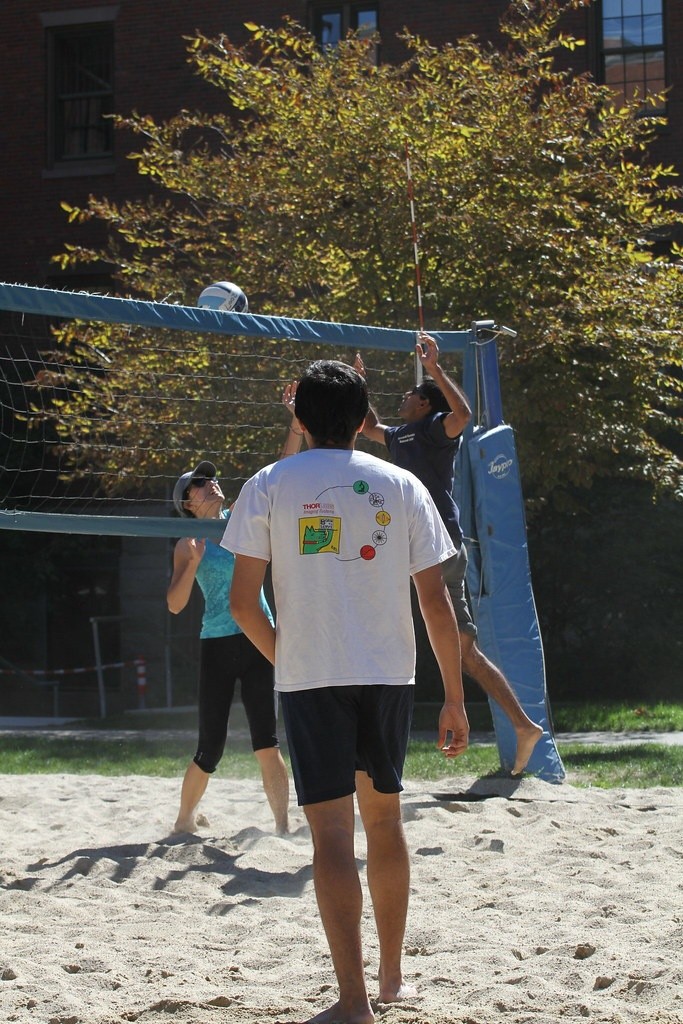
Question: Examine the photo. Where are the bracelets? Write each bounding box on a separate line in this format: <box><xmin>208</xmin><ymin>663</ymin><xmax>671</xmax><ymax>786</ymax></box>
<box><xmin>289</xmin><ymin>425</ymin><xmax>303</xmax><ymax>436</ymax></box>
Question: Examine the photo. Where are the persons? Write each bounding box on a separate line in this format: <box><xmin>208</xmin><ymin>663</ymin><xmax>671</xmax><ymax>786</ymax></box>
<box><xmin>166</xmin><ymin>380</ymin><xmax>305</xmax><ymax>838</ymax></box>
<box><xmin>219</xmin><ymin>360</ymin><xmax>470</xmax><ymax>1024</ymax></box>
<box><xmin>352</xmin><ymin>336</ymin><xmax>543</xmax><ymax>776</ymax></box>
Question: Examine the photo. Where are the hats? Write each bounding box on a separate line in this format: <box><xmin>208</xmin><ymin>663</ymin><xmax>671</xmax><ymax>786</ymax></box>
<box><xmin>173</xmin><ymin>461</ymin><xmax>217</xmax><ymax>517</ymax></box>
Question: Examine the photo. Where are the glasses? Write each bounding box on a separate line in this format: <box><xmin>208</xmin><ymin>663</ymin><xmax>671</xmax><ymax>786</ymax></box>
<box><xmin>185</xmin><ymin>479</ymin><xmax>218</xmax><ymax>498</ymax></box>
<box><xmin>412</xmin><ymin>386</ymin><xmax>428</xmax><ymax>399</ymax></box>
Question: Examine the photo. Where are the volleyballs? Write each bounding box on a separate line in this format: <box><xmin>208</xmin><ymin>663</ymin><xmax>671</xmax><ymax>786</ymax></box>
<box><xmin>199</xmin><ymin>281</ymin><xmax>250</xmax><ymax>312</ymax></box>
